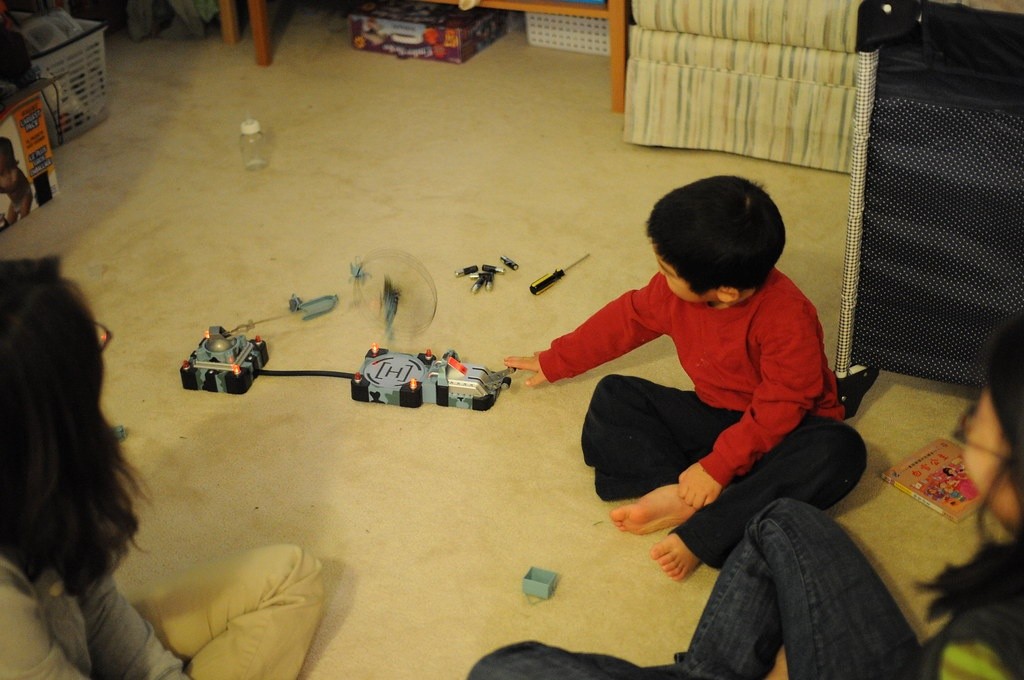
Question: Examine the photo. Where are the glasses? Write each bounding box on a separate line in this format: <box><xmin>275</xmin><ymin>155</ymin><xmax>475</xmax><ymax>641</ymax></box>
<box><xmin>96</xmin><ymin>322</ymin><xmax>113</xmax><ymax>354</ymax></box>
<box><xmin>953</xmin><ymin>405</ymin><xmax>1009</xmax><ymax>462</ymax></box>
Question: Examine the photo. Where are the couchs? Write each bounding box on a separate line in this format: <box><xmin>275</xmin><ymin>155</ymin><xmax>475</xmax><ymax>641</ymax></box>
<box><xmin>608</xmin><ymin>0</ymin><xmax>863</xmax><ymax>173</ymax></box>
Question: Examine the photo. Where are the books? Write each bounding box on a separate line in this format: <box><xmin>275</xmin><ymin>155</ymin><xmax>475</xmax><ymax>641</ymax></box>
<box><xmin>882</xmin><ymin>438</ymin><xmax>985</xmax><ymax>523</ymax></box>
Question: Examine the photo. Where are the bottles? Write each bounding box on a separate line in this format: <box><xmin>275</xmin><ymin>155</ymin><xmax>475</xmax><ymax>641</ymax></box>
<box><xmin>238</xmin><ymin>119</ymin><xmax>268</xmax><ymax>172</ymax></box>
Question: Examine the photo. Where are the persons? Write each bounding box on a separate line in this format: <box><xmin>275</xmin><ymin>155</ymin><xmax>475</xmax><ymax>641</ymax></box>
<box><xmin>1</xmin><ymin>253</ymin><xmax>329</xmax><ymax>680</ymax></box>
<box><xmin>466</xmin><ymin>174</ymin><xmax>1024</xmax><ymax>680</ymax></box>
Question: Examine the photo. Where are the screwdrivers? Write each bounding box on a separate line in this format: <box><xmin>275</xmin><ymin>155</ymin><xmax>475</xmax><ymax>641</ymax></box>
<box><xmin>530</xmin><ymin>254</ymin><xmax>589</xmax><ymax>295</ymax></box>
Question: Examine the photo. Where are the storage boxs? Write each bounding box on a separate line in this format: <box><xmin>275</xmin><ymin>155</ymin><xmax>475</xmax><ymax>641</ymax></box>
<box><xmin>0</xmin><ymin>92</ymin><xmax>59</xmax><ymax>232</ymax></box>
<box><xmin>348</xmin><ymin>2</ymin><xmax>501</xmax><ymax>64</ymax></box>
<box><xmin>522</xmin><ymin>13</ymin><xmax>611</xmax><ymax>58</ymax></box>
<box><xmin>7</xmin><ymin>12</ymin><xmax>107</xmax><ymax>143</ymax></box>
